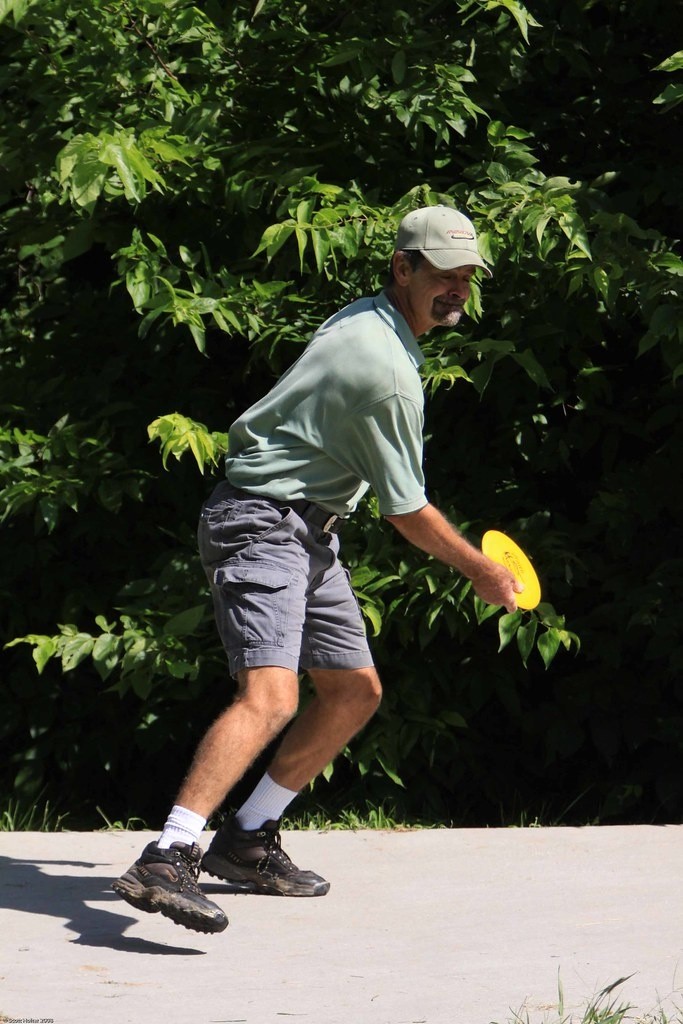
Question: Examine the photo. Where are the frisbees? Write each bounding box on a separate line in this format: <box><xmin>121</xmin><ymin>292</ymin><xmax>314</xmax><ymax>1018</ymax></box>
<box><xmin>482</xmin><ymin>529</ymin><xmax>541</xmax><ymax>610</ymax></box>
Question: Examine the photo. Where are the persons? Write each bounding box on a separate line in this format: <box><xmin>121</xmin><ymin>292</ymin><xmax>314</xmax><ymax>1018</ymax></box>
<box><xmin>112</xmin><ymin>200</ymin><xmax>526</xmax><ymax>936</ymax></box>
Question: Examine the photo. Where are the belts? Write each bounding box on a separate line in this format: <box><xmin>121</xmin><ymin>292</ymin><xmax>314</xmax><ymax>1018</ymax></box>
<box><xmin>288</xmin><ymin>499</ymin><xmax>346</xmax><ymax>533</ymax></box>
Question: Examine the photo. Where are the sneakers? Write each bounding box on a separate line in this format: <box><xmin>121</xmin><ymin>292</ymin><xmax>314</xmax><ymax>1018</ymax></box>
<box><xmin>110</xmin><ymin>841</ymin><xmax>228</xmax><ymax>934</ymax></box>
<box><xmin>199</xmin><ymin>809</ymin><xmax>329</xmax><ymax>895</ymax></box>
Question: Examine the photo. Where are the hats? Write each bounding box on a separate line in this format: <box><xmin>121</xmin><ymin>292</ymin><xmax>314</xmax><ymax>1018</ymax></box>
<box><xmin>396</xmin><ymin>205</ymin><xmax>492</xmax><ymax>278</ymax></box>
